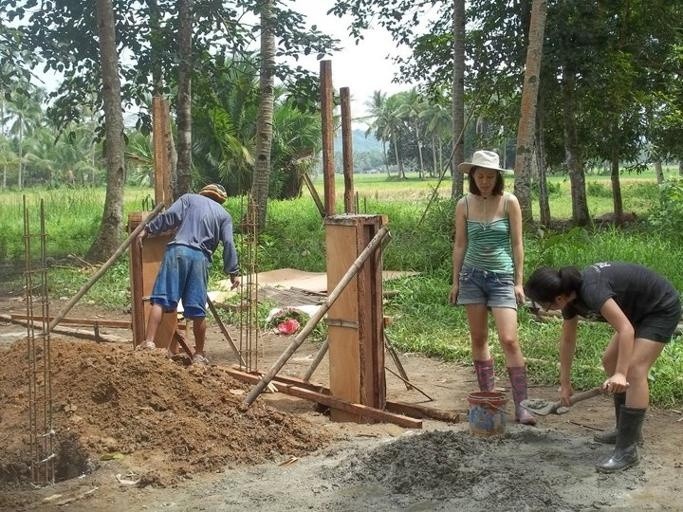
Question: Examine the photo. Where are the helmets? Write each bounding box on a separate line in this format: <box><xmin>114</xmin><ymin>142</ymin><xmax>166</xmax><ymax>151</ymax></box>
<box><xmin>198</xmin><ymin>183</ymin><xmax>228</xmax><ymax>204</ymax></box>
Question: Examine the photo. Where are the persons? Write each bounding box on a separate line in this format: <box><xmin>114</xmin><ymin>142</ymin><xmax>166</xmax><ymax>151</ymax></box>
<box><xmin>136</xmin><ymin>183</ymin><xmax>240</xmax><ymax>365</ymax></box>
<box><xmin>525</xmin><ymin>260</ymin><xmax>681</xmax><ymax>472</ymax></box>
<box><xmin>450</xmin><ymin>150</ymin><xmax>535</xmax><ymax>425</ymax></box>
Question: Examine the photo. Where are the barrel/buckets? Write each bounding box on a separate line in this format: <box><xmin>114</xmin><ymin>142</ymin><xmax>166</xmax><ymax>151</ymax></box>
<box><xmin>467</xmin><ymin>392</ymin><xmax>508</xmax><ymax>433</ymax></box>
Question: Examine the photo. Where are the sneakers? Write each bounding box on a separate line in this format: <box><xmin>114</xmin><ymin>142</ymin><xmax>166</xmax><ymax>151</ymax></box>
<box><xmin>193</xmin><ymin>353</ymin><xmax>210</xmax><ymax>364</ymax></box>
<box><xmin>135</xmin><ymin>340</ymin><xmax>156</xmax><ymax>350</ymax></box>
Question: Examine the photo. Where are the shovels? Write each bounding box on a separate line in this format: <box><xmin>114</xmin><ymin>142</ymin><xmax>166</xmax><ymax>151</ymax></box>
<box><xmin>520</xmin><ymin>381</ymin><xmax>630</xmax><ymax>416</ymax></box>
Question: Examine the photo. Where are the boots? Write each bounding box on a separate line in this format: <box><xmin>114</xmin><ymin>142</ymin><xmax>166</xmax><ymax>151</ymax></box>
<box><xmin>473</xmin><ymin>358</ymin><xmax>497</xmax><ymax>391</ymax></box>
<box><xmin>593</xmin><ymin>391</ymin><xmax>644</xmax><ymax>445</ymax></box>
<box><xmin>595</xmin><ymin>402</ymin><xmax>647</xmax><ymax>474</ymax></box>
<box><xmin>506</xmin><ymin>363</ymin><xmax>537</xmax><ymax>425</ymax></box>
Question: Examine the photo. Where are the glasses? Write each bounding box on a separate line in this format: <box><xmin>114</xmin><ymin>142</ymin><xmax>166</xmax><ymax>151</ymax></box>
<box><xmin>542</xmin><ymin>301</ymin><xmax>552</xmax><ymax>312</ymax></box>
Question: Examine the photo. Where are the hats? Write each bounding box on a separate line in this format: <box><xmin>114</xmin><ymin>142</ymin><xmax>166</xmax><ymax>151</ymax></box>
<box><xmin>457</xmin><ymin>149</ymin><xmax>514</xmax><ymax>177</ymax></box>
